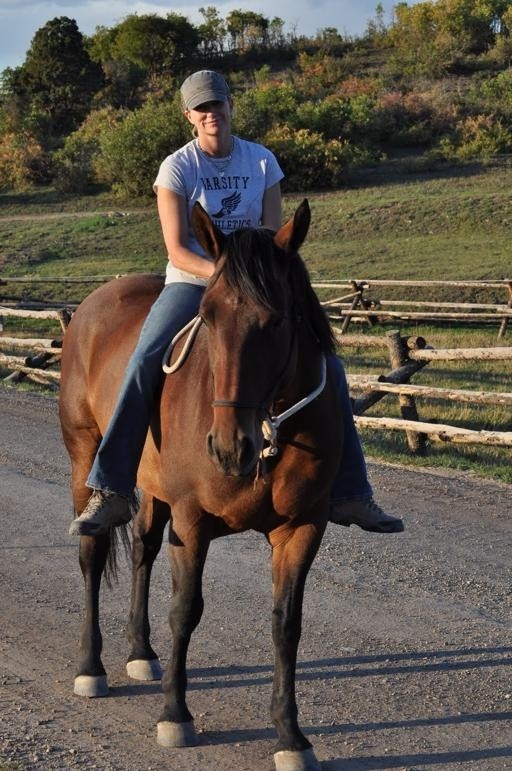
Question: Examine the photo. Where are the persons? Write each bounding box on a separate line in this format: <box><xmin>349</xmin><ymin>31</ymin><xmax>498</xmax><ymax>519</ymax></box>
<box><xmin>67</xmin><ymin>68</ymin><xmax>404</xmax><ymax>537</ymax></box>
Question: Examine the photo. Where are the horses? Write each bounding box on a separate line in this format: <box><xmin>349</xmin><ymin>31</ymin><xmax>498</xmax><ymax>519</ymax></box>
<box><xmin>59</xmin><ymin>198</ymin><xmax>344</xmax><ymax>771</ymax></box>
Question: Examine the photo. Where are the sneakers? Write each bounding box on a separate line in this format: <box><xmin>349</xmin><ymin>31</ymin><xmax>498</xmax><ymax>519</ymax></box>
<box><xmin>70</xmin><ymin>490</ymin><xmax>131</xmax><ymax>536</ymax></box>
<box><xmin>329</xmin><ymin>496</ymin><xmax>403</xmax><ymax>533</ymax></box>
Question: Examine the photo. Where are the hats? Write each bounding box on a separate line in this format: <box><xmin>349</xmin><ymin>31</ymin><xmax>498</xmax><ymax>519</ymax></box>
<box><xmin>180</xmin><ymin>70</ymin><xmax>231</xmax><ymax>110</ymax></box>
